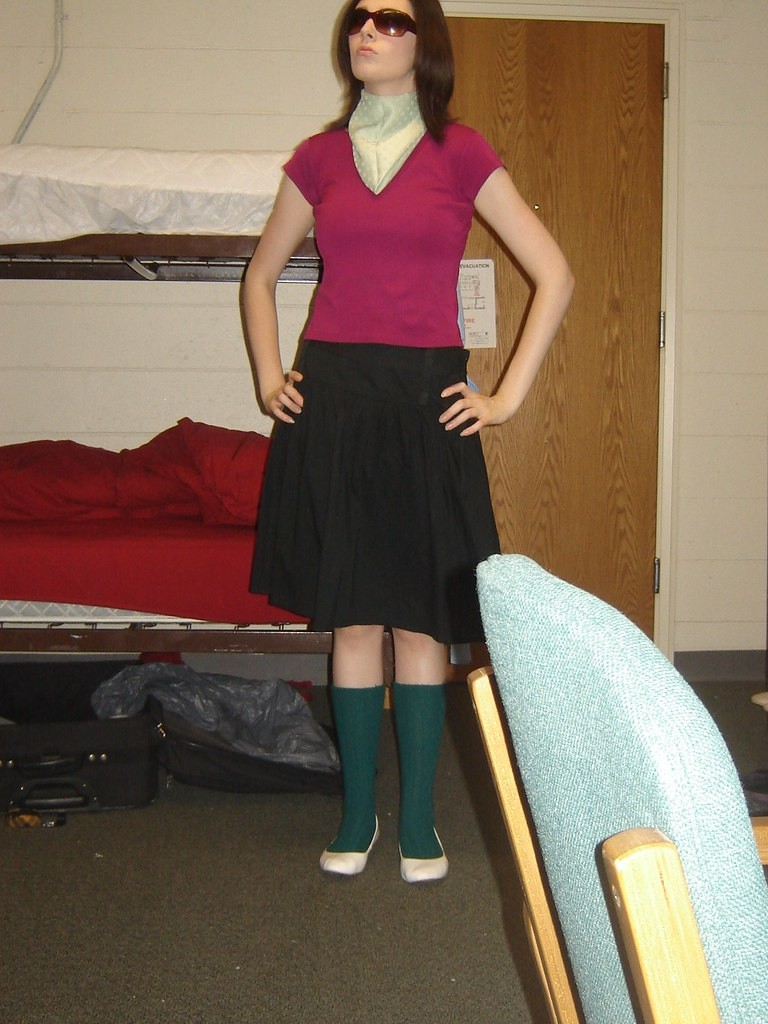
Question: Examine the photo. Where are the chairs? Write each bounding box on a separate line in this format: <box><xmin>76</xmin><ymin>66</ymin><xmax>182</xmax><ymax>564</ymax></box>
<box><xmin>464</xmin><ymin>554</ymin><xmax>768</xmax><ymax>1023</ymax></box>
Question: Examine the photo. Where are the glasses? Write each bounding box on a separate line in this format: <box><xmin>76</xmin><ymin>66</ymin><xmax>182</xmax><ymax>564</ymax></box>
<box><xmin>348</xmin><ymin>7</ymin><xmax>417</xmax><ymax>37</ymax></box>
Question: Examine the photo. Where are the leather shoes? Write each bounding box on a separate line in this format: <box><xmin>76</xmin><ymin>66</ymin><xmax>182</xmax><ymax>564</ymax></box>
<box><xmin>398</xmin><ymin>826</ymin><xmax>449</xmax><ymax>883</ymax></box>
<box><xmin>319</xmin><ymin>813</ymin><xmax>379</xmax><ymax>875</ymax></box>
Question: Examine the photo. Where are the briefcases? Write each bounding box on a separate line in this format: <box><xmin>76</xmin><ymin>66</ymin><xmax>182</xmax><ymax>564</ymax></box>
<box><xmin>0</xmin><ymin>664</ymin><xmax>165</xmax><ymax>828</ymax></box>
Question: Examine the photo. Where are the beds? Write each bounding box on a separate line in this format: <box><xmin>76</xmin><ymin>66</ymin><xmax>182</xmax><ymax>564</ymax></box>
<box><xmin>0</xmin><ymin>145</ymin><xmax>323</xmax><ymax>284</ymax></box>
<box><xmin>1</xmin><ymin>519</ymin><xmax>395</xmax><ymax>691</ymax></box>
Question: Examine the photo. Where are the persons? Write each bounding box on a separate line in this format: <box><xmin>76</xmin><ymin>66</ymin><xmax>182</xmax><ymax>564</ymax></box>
<box><xmin>241</xmin><ymin>0</ymin><xmax>575</xmax><ymax>882</ymax></box>
